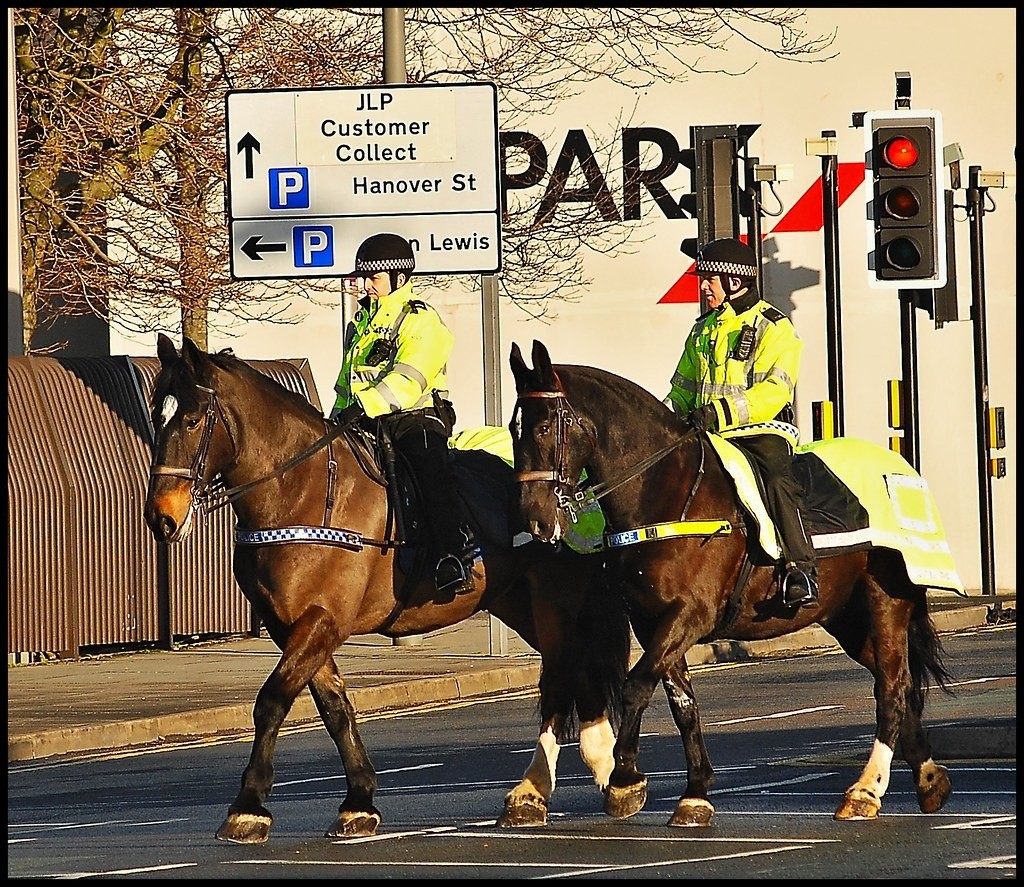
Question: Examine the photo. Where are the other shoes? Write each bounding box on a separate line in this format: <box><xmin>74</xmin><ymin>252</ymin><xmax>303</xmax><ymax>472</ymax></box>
<box><xmin>436</xmin><ymin>563</ymin><xmax>475</xmax><ymax>595</ymax></box>
<box><xmin>787</xmin><ymin>568</ymin><xmax>820</xmax><ymax>609</ymax></box>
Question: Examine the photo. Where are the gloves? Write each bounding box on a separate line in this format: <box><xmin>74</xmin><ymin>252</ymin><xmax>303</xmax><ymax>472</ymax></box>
<box><xmin>333</xmin><ymin>402</ymin><xmax>365</xmax><ymax>426</ymax></box>
<box><xmin>687</xmin><ymin>404</ymin><xmax>717</xmax><ymax>432</ymax></box>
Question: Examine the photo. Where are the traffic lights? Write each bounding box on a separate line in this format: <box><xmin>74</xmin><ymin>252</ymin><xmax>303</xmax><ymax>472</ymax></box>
<box><xmin>680</xmin><ymin>123</ymin><xmax>740</xmax><ymax>265</ymax></box>
<box><xmin>864</xmin><ymin>109</ymin><xmax>947</xmax><ymax>292</ymax></box>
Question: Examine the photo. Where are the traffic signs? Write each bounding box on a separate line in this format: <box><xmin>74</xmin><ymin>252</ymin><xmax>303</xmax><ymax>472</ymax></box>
<box><xmin>225</xmin><ymin>80</ymin><xmax>502</xmax><ymax>220</ymax></box>
<box><xmin>228</xmin><ymin>210</ymin><xmax>502</xmax><ymax>278</ymax></box>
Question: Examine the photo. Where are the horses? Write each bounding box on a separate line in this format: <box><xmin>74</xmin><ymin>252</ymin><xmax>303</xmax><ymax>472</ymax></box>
<box><xmin>141</xmin><ymin>330</ymin><xmax>633</xmax><ymax>844</ymax></box>
<box><xmin>491</xmin><ymin>337</ymin><xmax>963</xmax><ymax>832</ymax></box>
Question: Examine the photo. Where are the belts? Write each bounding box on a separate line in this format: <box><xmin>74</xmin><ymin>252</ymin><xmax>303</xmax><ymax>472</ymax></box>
<box><xmin>384</xmin><ymin>407</ymin><xmax>437</xmax><ymax>423</ymax></box>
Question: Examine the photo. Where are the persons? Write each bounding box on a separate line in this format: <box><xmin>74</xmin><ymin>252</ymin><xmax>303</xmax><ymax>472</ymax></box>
<box><xmin>669</xmin><ymin>238</ymin><xmax>821</xmax><ymax>608</ymax></box>
<box><xmin>329</xmin><ymin>233</ymin><xmax>479</xmax><ymax>595</ymax></box>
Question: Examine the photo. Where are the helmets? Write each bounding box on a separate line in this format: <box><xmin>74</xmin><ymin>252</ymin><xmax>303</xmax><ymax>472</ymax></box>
<box><xmin>684</xmin><ymin>238</ymin><xmax>758</xmax><ymax>279</ymax></box>
<box><xmin>347</xmin><ymin>234</ymin><xmax>416</xmax><ymax>277</ymax></box>
